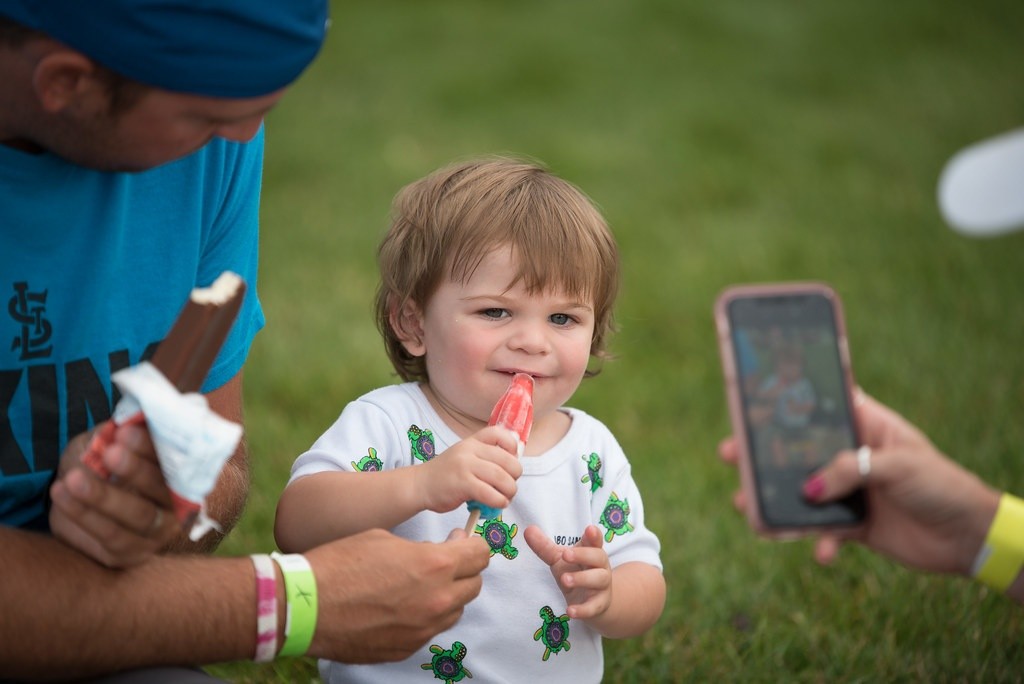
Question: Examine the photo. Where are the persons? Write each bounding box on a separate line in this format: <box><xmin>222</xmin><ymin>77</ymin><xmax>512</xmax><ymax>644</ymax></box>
<box><xmin>0</xmin><ymin>1</ymin><xmax>489</xmax><ymax>684</ymax></box>
<box><xmin>718</xmin><ymin>383</ymin><xmax>1024</xmax><ymax>611</ymax></box>
<box><xmin>736</xmin><ymin>330</ymin><xmax>760</xmax><ymax>400</ymax></box>
<box><xmin>757</xmin><ymin>349</ymin><xmax>817</xmax><ymax>471</ymax></box>
<box><xmin>272</xmin><ymin>153</ymin><xmax>665</xmax><ymax>684</ymax></box>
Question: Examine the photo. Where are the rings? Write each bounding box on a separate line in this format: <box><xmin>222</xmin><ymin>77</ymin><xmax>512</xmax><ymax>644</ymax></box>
<box><xmin>857</xmin><ymin>445</ymin><xmax>871</xmax><ymax>477</ymax></box>
<box><xmin>146</xmin><ymin>508</ymin><xmax>162</xmax><ymax>536</ymax></box>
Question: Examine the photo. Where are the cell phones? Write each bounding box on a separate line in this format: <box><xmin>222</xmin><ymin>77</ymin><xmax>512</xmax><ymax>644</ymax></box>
<box><xmin>714</xmin><ymin>282</ymin><xmax>876</xmax><ymax>541</ymax></box>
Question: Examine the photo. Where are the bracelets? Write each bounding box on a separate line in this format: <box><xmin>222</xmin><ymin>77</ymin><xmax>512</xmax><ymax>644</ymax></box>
<box><xmin>271</xmin><ymin>552</ymin><xmax>317</xmax><ymax>657</ymax></box>
<box><xmin>856</xmin><ymin>392</ymin><xmax>867</xmax><ymax>405</ymax></box>
<box><xmin>969</xmin><ymin>492</ymin><xmax>1024</xmax><ymax>595</ymax></box>
<box><xmin>249</xmin><ymin>553</ymin><xmax>279</xmax><ymax>664</ymax></box>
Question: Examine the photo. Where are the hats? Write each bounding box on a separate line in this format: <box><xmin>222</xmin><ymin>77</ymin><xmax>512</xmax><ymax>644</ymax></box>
<box><xmin>938</xmin><ymin>129</ymin><xmax>1024</xmax><ymax>237</ymax></box>
<box><xmin>2</xmin><ymin>0</ymin><xmax>332</xmax><ymax>98</ymax></box>
